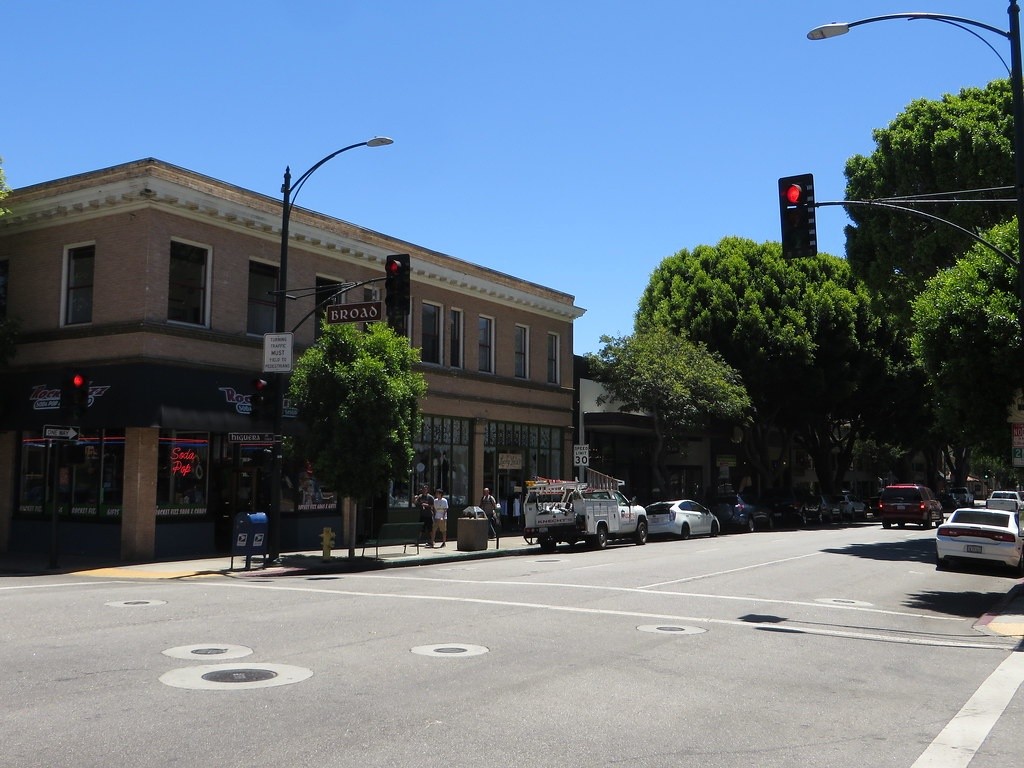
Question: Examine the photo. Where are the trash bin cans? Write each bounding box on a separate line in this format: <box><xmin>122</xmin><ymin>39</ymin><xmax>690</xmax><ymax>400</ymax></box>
<box><xmin>457</xmin><ymin>506</ymin><xmax>488</xmax><ymax>551</ymax></box>
<box><xmin>231</xmin><ymin>512</ymin><xmax>268</xmax><ymax>568</ymax></box>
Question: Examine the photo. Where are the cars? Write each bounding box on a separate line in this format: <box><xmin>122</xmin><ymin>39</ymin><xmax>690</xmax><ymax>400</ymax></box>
<box><xmin>644</xmin><ymin>500</ymin><xmax>721</xmax><ymax>541</ymax></box>
<box><xmin>935</xmin><ymin>507</ymin><xmax>1024</xmax><ymax>576</ymax></box>
<box><xmin>934</xmin><ymin>487</ymin><xmax>976</xmax><ymax>512</ymax></box>
<box><xmin>772</xmin><ymin>485</ymin><xmax>882</xmax><ymax>530</ymax></box>
<box><xmin>1014</xmin><ymin>490</ymin><xmax>1024</xmax><ymax>502</ymax></box>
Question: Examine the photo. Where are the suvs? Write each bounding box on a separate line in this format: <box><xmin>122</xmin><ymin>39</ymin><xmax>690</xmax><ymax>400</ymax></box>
<box><xmin>705</xmin><ymin>491</ymin><xmax>775</xmax><ymax>534</ymax></box>
<box><xmin>878</xmin><ymin>481</ymin><xmax>944</xmax><ymax>530</ymax></box>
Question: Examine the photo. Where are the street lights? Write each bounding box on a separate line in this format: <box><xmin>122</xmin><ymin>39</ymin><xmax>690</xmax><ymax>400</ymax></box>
<box><xmin>806</xmin><ymin>0</ymin><xmax>1024</xmax><ymax>420</ymax></box>
<box><xmin>268</xmin><ymin>133</ymin><xmax>395</xmax><ymax>559</ymax></box>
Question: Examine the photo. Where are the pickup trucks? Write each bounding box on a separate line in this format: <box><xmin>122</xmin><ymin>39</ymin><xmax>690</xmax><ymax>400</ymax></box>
<box><xmin>523</xmin><ymin>467</ymin><xmax>650</xmax><ymax>555</ymax></box>
<box><xmin>985</xmin><ymin>490</ymin><xmax>1023</xmax><ymax>513</ymax></box>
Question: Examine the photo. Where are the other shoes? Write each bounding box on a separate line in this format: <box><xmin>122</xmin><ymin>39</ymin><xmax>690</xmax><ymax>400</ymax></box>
<box><xmin>426</xmin><ymin>542</ymin><xmax>434</xmax><ymax>546</ymax></box>
<box><xmin>440</xmin><ymin>543</ymin><xmax>446</xmax><ymax>548</ymax></box>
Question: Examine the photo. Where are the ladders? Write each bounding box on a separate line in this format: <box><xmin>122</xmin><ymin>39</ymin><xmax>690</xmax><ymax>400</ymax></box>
<box><xmin>585</xmin><ymin>467</ymin><xmax>619</xmax><ymax>493</ymax></box>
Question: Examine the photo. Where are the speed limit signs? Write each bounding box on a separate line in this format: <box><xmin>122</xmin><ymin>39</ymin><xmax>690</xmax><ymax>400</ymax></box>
<box><xmin>573</xmin><ymin>444</ymin><xmax>590</xmax><ymax>467</ymax></box>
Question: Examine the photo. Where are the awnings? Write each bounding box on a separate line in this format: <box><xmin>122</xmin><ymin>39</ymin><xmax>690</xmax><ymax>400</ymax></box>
<box><xmin>0</xmin><ymin>362</ymin><xmax>311</xmax><ymax>434</ymax></box>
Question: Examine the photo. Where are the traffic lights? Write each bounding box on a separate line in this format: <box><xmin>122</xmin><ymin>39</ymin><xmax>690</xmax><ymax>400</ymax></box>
<box><xmin>62</xmin><ymin>368</ymin><xmax>84</xmax><ymax>399</ymax></box>
<box><xmin>778</xmin><ymin>173</ymin><xmax>817</xmax><ymax>259</ymax></box>
<box><xmin>383</xmin><ymin>253</ymin><xmax>412</xmax><ymax>317</ymax></box>
<box><xmin>253</xmin><ymin>369</ymin><xmax>276</xmax><ymax>398</ymax></box>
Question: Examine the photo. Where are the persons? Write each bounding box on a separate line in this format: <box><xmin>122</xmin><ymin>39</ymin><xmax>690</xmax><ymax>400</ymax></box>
<box><xmin>431</xmin><ymin>489</ymin><xmax>449</xmax><ymax>548</ymax></box>
<box><xmin>413</xmin><ymin>485</ymin><xmax>434</xmax><ymax>547</ymax></box>
<box><xmin>478</xmin><ymin>488</ymin><xmax>497</xmax><ymax>538</ymax></box>
